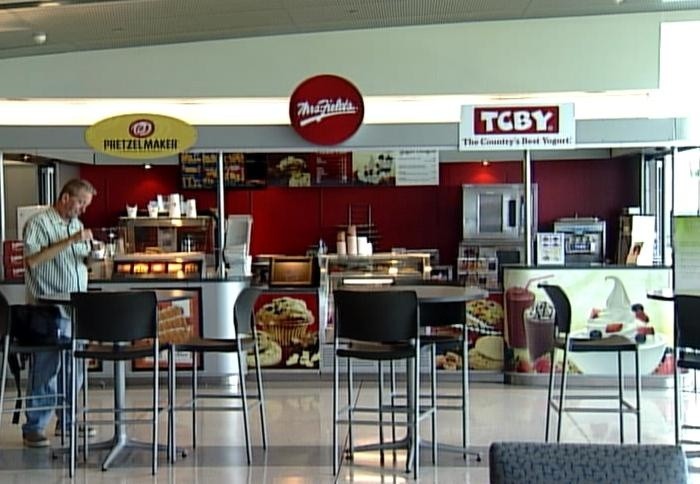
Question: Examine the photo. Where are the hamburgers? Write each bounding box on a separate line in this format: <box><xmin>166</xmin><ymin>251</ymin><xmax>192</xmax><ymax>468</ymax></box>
<box><xmin>468</xmin><ymin>336</ymin><xmax>506</xmax><ymax>369</ymax></box>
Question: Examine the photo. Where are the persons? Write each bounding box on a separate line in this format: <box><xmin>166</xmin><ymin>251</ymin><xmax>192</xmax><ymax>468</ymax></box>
<box><xmin>21</xmin><ymin>177</ymin><xmax>105</xmax><ymax>448</ymax></box>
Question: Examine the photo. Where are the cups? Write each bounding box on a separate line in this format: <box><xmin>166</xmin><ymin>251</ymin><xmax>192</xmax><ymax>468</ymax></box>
<box><xmin>335</xmin><ymin>224</ymin><xmax>357</xmax><ymax>256</ymax></box>
<box><xmin>505</xmin><ymin>291</ymin><xmax>535</xmax><ymax>348</ymax></box>
<box><xmin>125</xmin><ymin>191</ymin><xmax>199</xmax><ymax>218</ymax></box>
<box><xmin>91</xmin><ymin>241</ymin><xmax>104</xmax><ymax>259</ymax></box>
<box><xmin>524</xmin><ymin>316</ymin><xmax>555</xmax><ymax>365</ymax></box>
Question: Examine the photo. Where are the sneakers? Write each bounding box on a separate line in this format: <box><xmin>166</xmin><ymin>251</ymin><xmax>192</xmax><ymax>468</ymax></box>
<box><xmin>55</xmin><ymin>426</ymin><xmax>98</xmax><ymax>436</ymax></box>
<box><xmin>22</xmin><ymin>432</ymin><xmax>49</xmax><ymax>447</ymax></box>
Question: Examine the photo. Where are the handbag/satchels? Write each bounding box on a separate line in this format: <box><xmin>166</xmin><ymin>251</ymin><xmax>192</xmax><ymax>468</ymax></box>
<box><xmin>8</xmin><ymin>304</ymin><xmax>63</xmax><ymax>348</ymax></box>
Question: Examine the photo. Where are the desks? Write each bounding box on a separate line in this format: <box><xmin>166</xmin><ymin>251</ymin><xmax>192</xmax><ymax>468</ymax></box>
<box><xmin>333</xmin><ymin>283</ymin><xmax>488</xmax><ymax>466</ymax></box>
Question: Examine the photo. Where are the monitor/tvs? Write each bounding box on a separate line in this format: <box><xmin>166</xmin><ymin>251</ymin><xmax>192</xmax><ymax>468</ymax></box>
<box><xmin>269</xmin><ymin>256</ymin><xmax>314</xmax><ymax>289</ymax></box>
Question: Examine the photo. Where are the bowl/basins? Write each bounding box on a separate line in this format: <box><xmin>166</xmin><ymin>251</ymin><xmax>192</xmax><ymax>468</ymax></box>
<box><xmin>566</xmin><ymin>330</ymin><xmax>669</xmax><ymax>376</ymax></box>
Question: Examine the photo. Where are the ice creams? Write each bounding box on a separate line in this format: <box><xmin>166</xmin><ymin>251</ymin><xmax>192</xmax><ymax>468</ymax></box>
<box><xmin>587</xmin><ymin>274</ymin><xmax>655</xmax><ymax>349</ymax></box>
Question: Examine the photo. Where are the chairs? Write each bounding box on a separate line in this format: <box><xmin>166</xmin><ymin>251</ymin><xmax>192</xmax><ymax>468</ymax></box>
<box><xmin>489</xmin><ymin>441</ymin><xmax>687</xmax><ymax>484</ymax></box>
<box><xmin>536</xmin><ymin>282</ymin><xmax>700</xmax><ymax>448</ymax></box>
<box><xmin>0</xmin><ymin>284</ymin><xmax>266</xmax><ymax>478</ymax></box>
<box><xmin>333</xmin><ymin>275</ymin><xmax>470</xmax><ymax>481</ymax></box>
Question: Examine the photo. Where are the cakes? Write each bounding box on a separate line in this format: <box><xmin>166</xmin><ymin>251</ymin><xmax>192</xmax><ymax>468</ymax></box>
<box><xmin>255</xmin><ymin>296</ymin><xmax>316</xmax><ymax>346</ymax></box>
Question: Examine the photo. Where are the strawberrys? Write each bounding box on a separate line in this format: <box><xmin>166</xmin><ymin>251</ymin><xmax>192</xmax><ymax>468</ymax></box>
<box><xmin>516</xmin><ymin>360</ymin><xmax>530</xmax><ymax>372</ymax></box>
<box><xmin>533</xmin><ymin>352</ymin><xmax>551</xmax><ymax>373</ymax></box>
